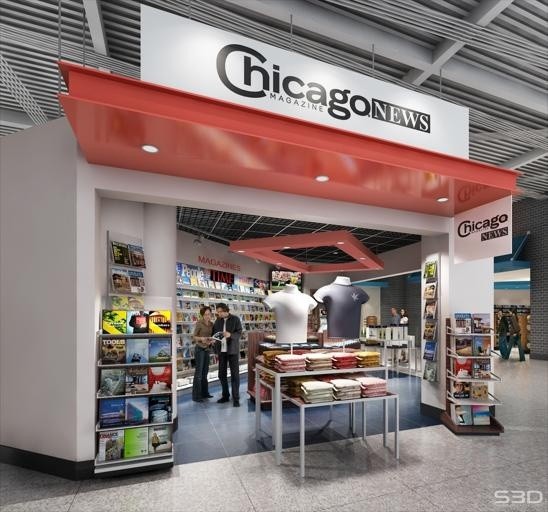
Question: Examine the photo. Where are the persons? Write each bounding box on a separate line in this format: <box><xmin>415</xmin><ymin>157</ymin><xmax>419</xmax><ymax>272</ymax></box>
<box><xmin>208</xmin><ymin>303</ymin><xmax>243</xmax><ymax>407</ymax></box>
<box><xmin>497</xmin><ymin>310</ymin><xmax>509</xmax><ymax>359</ymax></box>
<box><xmin>389</xmin><ymin>307</ymin><xmax>401</xmax><ymax>325</ymax></box>
<box><xmin>503</xmin><ymin>308</ymin><xmax>525</xmax><ymax>360</ymax></box>
<box><xmin>313</xmin><ymin>275</ymin><xmax>369</xmax><ymax>337</ymax></box>
<box><xmin>397</xmin><ymin>307</ymin><xmax>409</xmax><ymax>326</ymax></box>
<box><xmin>191</xmin><ymin>306</ymin><xmax>215</xmax><ymax>402</ymax></box>
<box><xmin>261</xmin><ymin>283</ymin><xmax>318</xmax><ymax>344</ymax></box>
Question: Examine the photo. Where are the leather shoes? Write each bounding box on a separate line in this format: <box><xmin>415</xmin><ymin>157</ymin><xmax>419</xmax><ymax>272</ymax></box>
<box><xmin>192</xmin><ymin>393</ymin><xmax>240</xmax><ymax>408</ymax></box>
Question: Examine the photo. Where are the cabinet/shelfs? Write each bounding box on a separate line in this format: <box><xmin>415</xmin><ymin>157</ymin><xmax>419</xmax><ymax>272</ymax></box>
<box><xmin>444</xmin><ymin>318</ymin><xmax>505</xmax><ymax>434</ymax></box>
<box><xmin>94</xmin><ymin>285</ymin><xmax>399</xmax><ymax>479</ymax></box>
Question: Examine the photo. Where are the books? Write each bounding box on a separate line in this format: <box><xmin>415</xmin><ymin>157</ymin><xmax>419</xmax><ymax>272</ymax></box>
<box><xmin>453</xmin><ymin>311</ymin><xmax>494</xmax><ymax>427</ymax></box>
<box><xmin>420</xmin><ymin>259</ymin><xmax>439</xmax><ymax>383</ymax></box>
<box><xmin>96</xmin><ymin>241</ymin><xmax>173</xmax><ymax>463</ymax></box>
